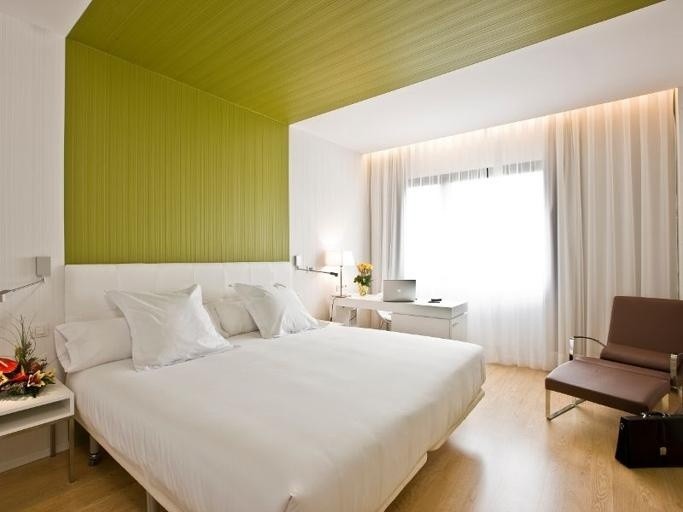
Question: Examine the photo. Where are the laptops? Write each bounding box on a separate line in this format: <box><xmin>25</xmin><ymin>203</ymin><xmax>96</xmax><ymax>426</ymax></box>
<box><xmin>383</xmin><ymin>280</ymin><xmax>416</xmax><ymax>302</ymax></box>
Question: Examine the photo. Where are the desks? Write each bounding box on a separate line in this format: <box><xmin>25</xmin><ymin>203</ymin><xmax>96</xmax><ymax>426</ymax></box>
<box><xmin>328</xmin><ymin>293</ymin><xmax>469</xmax><ymax>343</ymax></box>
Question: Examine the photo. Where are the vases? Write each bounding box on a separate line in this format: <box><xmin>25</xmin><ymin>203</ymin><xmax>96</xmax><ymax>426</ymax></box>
<box><xmin>359</xmin><ymin>285</ymin><xmax>368</xmax><ymax>296</ymax></box>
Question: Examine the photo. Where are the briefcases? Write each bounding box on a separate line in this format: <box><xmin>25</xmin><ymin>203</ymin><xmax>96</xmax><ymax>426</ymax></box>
<box><xmin>615</xmin><ymin>411</ymin><xmax>683</xmax><ymax>468</ymax></box>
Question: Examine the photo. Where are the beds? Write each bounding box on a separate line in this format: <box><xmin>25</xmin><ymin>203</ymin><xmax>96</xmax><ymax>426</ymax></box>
<box><xmin>58</xmin><ymin>262</ymin><xmax>486</xmax><ymax>511</ymax></box>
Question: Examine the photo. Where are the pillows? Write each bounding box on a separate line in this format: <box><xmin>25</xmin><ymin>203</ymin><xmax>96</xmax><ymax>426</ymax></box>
<box><xmin>208</xmin><ymin>289</ymin><xmax>257</xmax><ymax>336</ymax></box>
<box><xmin>228</xmin><ymin>278</ymin><xmax>319</xmax><ymax>339</ymax></box>
<box><xmin>104</xmin><ymin>282</ymin><xmax>234</xmax><ymax>373</ymax></box>
<box><xmin>54</xmin><ymin>304</ymin><xmax>228</xmax><ymax>374</ymax></box>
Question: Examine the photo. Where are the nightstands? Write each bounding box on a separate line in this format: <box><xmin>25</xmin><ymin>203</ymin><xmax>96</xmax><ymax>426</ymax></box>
<box><xmin>0</xmin><ymin>375</ymin><xmax>76</xmax><ymax>483</ymax></box>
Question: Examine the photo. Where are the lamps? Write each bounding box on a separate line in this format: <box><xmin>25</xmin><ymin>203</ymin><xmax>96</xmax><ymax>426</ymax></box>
<box><xmin>294</xmin><ymin>255</ymin><xmax>338</xmax><ymax>277</ymax></box>
<box><xmin>324</xmin><ymin>250</ymin><xmax>355</xmax><ymax>298</ymax></box>
<box><xmin>0</xmin><ymin>256</ymin><xmax>51</xmax><ymax>302</ymax></box>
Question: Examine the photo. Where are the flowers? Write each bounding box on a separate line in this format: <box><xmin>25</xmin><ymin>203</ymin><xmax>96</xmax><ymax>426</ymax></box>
<box><xmin>0</xmin><ymin>310</ymin><xmax>54</xmax><ymax>398</ymax></box>
<box><xmin>353</xmin><ymin>262</ymin><xmax>376</xmax><ymax>287</ymax></box>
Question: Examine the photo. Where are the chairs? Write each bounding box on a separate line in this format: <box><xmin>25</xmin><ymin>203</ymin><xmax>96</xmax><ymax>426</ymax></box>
<box><xmin>375</xmin><ymin>293</ymin><xmax>392</xmax><ymax>331</ymax></box>
<box><xmin>543</xmin><ymin>296</ymin><xmax>683</xmax><ymax>421</ymax></box>
